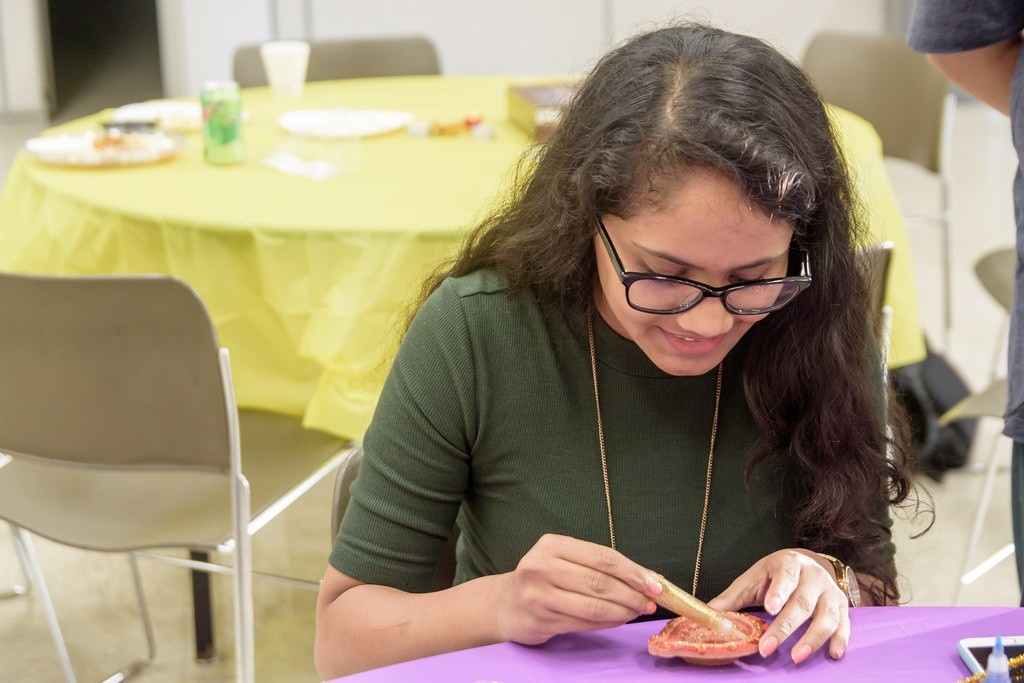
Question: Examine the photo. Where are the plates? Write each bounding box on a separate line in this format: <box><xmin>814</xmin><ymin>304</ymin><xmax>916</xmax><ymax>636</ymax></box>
<box><xmin>114</xmin><ymin>99</ymin><xmax>203</xmax><ymax>129</ymax></box>
<box><xmin>24</xmin><ymin>133</ymin><xmax>176</xmax><ymax>167</ymax></box>
<box><xmin>276</xmin><ymin>109</ymin><xmax>414</xmax><ymax>140</ymax></box>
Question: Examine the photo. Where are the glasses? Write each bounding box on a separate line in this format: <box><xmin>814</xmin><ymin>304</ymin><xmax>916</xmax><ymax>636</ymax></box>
<box><xmin>591</xmin><ymin>207</ymin><xmax>815</xmax><ymax>317</ymax></box>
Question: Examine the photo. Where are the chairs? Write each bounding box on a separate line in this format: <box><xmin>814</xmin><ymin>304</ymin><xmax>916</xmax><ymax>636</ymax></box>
<box><xmin>972</xmin><ymin>246</ymin><xmax>1019</xmax><ymax>385</ymax></box>
<box><xmin>1</xmin><ymin>271</ymin><xmax>362</xmax><ymax>683</ymax></box>
<box><xmin>233</xmin><ymin>36</ymin><xmax>439</xmax><ymax>91</ymax></box>
<box><xmin>936</xmin><ymin>379</ymin><xmax>1017</xmax><ymax>608</ymax></box>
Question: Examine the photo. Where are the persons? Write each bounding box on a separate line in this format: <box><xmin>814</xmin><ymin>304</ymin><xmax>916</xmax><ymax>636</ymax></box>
<box><xmin>902</xmin><ymin>0</ymin><xmax>1023</xmax><ymax>608</ymax></box>
<box><xmin>313</xmin><ymin>17</ymin><xmax>936</xmax><ymax>683</ymax></box>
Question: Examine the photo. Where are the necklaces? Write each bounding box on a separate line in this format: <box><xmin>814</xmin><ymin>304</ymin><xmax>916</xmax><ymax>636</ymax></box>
<box><xmin>585</xmin><ymin>284</ymin><xmax>724</xmax><ymax>598</ymax></box>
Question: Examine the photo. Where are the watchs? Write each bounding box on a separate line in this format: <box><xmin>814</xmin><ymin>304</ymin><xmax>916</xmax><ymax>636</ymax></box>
<box><xmin>816</xmin><ymin>552</ymin><xmax>861</xmax><ymax>607</ymax></box>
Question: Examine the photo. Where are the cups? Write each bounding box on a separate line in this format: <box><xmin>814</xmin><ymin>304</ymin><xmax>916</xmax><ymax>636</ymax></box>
<box><xmin>259</xmin><ymin>40</ymin><xmax>315</xmax><ymax>99</ymax></box>
<box><xmin>201</xmin><ymin>81</ymin><xmax>243</xmax><ymax>165</ymax></box>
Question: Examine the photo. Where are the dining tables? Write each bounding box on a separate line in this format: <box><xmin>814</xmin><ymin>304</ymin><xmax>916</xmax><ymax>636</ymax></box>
<box><xmin>315</xmin><ymin>604</ymin><xmax>1024</xmax><ymax>681</ymax></box>
<box><xmin>0</xmin><ymin>75</ymin><xmax>936</xmax><ymax>663</ymax></box>
<box><xmin>796</xmin><ymin>29</ymin><xmax>953</xmax><ymax>358</ymax></box>
